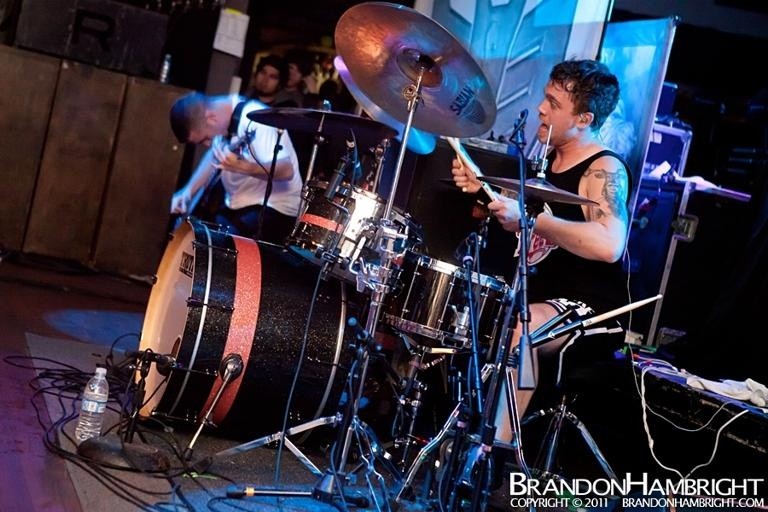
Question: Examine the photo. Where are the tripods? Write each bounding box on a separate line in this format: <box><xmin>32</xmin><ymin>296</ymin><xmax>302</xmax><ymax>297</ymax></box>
<box><xmin>394</xmin><ymin>218</ymin><xmax>536</xmax><ymax>512</ymax></box>
<box><xmin>347</xmin><ymin>381</ymin><xmax>429</xmax><ymax>500</ymax></box>
<box><xmin>191</xmin><ymin>289</ymin><xmax>416</xmax><ymax>512</ymax></box>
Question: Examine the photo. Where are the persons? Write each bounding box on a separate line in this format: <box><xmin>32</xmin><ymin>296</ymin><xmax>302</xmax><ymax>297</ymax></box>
<box><xmin>243</xmin><ymin>49</ymin><xmax>314</xmax><ymax>130</ymax></box>
<box><xmin>305</xmin><ymin>80</ymin><xmax>339</xmax><ymax>123</ymax></box>
<box><xmin>170</xmin><ymin>91</ymin><xmax>303</xmax><ymax>237</ymax></box>
<box><xmin>451</xmin><ymin>59</ymin><xmax>631</xmax><ymax>444</ymax></box>
<box><xmin>244</xmin><ymin>53</ymin><xmax>303</xmax><ymax>132</ymax></box>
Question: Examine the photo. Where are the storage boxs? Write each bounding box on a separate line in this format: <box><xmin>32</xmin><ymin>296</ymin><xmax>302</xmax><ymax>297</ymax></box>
<box><xmin>15</xmin><ymin>0</ymin><xmax>169</xmax><ymax>79</ymax></box>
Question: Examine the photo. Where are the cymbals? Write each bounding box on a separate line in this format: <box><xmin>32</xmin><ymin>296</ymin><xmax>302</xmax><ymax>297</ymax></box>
<box><xmin>332</xmin><ymin>55</ymin><xmax>437</xmax><ymax>155</ymax></box>
<box><xmin>335</xmin><ymin>3</ymin><xmax>497</xmax><ymax>138</ymax></box>
<box><xmin>247</xmin><ymin>108</ymin><xmax>400</xmax><ymax>139</ymax></box>
<box><xmin>477</xmin><ymin>174</ymin><xmax>601</xmax><ymax>207</ymax></box>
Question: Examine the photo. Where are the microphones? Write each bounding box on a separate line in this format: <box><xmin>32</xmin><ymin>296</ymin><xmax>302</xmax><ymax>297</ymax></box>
<box><xmin>453</xmin><ymin>233</ymin><xmax>475</xmax><ymax>261</ymax></box>
<box><xmin>123</xmin><ymin>348</ymin><xmax>173</xmax><ymax>377</ymax></box>
<box><xmin>346</xmin><ymin>127</ymin><xmax>362</xmax><ymax>180</ymax></box>
<box><xmin>512</xmin><ymin>111</ymin><xmax>526</xmax><ymax>139</ymax></box>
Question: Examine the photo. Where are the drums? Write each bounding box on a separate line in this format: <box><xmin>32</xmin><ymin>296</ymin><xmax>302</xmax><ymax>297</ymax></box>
<box><xmin>136</xmin><ymin>215</ymin><xmax>396</xmax><ymax>446</ymax></box>
<box><xmin>285</xmin><ymin>181</ymin><xmax>420</xmax><ymax>288</ymax></box>
<box><xmin>380</xmin><ymin>248</ymin><xmax>502</xmax><ymax>352</ymax></box>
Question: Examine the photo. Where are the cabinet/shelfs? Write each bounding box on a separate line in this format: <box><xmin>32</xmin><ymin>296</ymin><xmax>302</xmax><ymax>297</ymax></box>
<box><xmin>86</xmin><ymin>75</ymin><xmax>198</xmax><ymax>278</ymax></box>
<box><xmin>0</xmin><ymin>43</ymin><xmax>128</xmax><ymax>267</ymax></box>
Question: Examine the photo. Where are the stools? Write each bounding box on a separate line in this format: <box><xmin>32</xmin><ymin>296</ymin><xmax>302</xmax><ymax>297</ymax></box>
<box><xmin>520</xmin><ymin>351</ymin><xmax>625</xmax><ymax>502</ymax></box>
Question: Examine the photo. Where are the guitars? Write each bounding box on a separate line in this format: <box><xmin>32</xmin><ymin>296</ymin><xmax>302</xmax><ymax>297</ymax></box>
<box><xmin>150</xmin><ymin>128</ymin><xmax>257</xmax><ymax>262</ymax></box>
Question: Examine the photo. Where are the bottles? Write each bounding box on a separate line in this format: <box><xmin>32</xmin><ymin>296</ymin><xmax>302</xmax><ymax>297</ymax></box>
<box><xmin>75</xmin><ymin>368</ymin><xmax>109</xmax><ymax>442</ymax></box>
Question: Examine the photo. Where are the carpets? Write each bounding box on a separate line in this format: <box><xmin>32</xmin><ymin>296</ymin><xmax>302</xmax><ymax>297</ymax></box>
<box><xmin>24</xmin><ymin>331</ymin><xmax>569</xmax><ymax>511</ymax></box>
